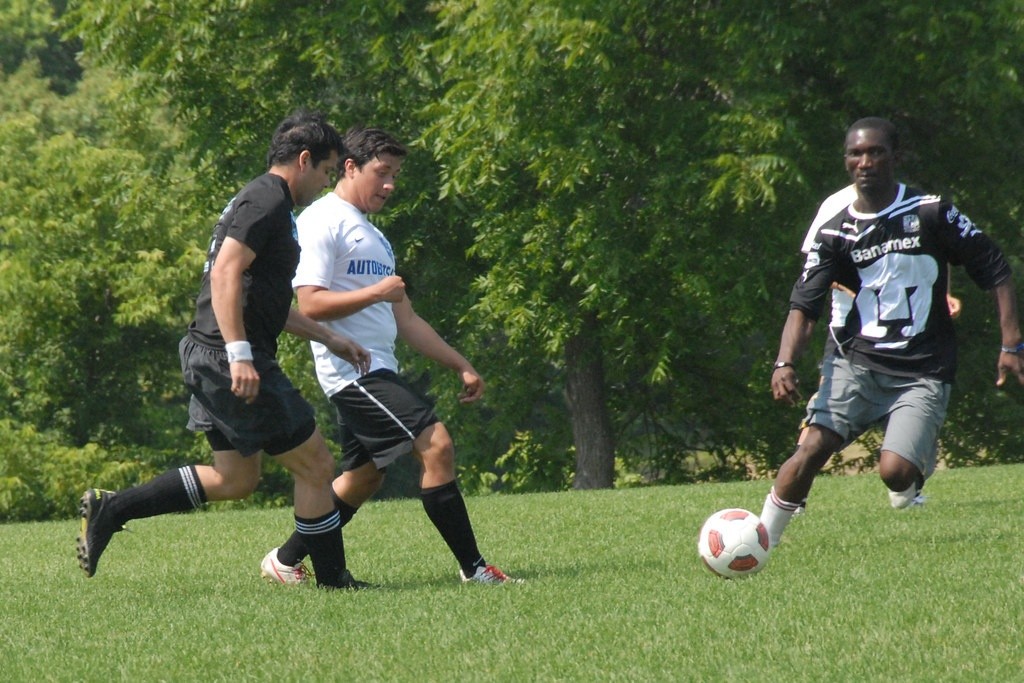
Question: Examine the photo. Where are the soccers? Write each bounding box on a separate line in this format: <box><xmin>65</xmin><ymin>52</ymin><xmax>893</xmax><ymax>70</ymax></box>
<box><xmin>698</xmin><ymin>508</ymin><xmax>770</xmax><ymax>580</ymax></box>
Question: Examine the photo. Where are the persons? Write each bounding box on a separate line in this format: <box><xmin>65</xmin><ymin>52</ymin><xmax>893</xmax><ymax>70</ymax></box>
<box><xmin>259</xmin><ymin>125</ymin><xmax>524</xmax><ymax>587</ymax></box>
<box><xmin>75</xmin><ymin>112</ymin><xmax>376</xmax><ymax>590</ymax></box>
<box><xmin>759</xmin><ymin>117</ymin><xmax>1024</xmax><ymax>554</ymax></box>
<box><xmin>793</xmin><ymin>182</ymin><xmax>962</xmax><ymax>515</ymax></box>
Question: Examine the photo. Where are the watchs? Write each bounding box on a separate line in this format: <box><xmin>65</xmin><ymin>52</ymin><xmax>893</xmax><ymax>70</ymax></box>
<box><xmin>773</xmin><ymin>362</ymin><xmax>795</xmax><ymax>369</ymax></box>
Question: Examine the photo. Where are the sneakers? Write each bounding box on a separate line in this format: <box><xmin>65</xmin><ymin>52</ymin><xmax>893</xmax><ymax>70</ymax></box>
<box><xmin>76</xmin><ymin>489</ymin><xmax>125</xmax><ymax>578</ymax></box>
<box><xmin>316</xmin><ymin>570</ymin><xmax>371</xmax><ymax>591</ymax></box>
<box><xmin>460</xmin><ymin>564</ymin><xmax>525</xmax><ymax>585</ymax></box>
<box><xmin>261</xmin><ymin>547</ymin><xmax>312</xmax><ymax>585</ymax></box>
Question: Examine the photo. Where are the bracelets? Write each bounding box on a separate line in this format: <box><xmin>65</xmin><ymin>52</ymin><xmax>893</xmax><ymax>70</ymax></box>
<box><xmin>225</xmin><ymin>341</ymin><xmax>254</xmax><ymax>362</ymax></box>
<box><xmin>1002</xmin><ymin>343</ymin><xmax>1024</xmax><ymax>352</ymax></box>
